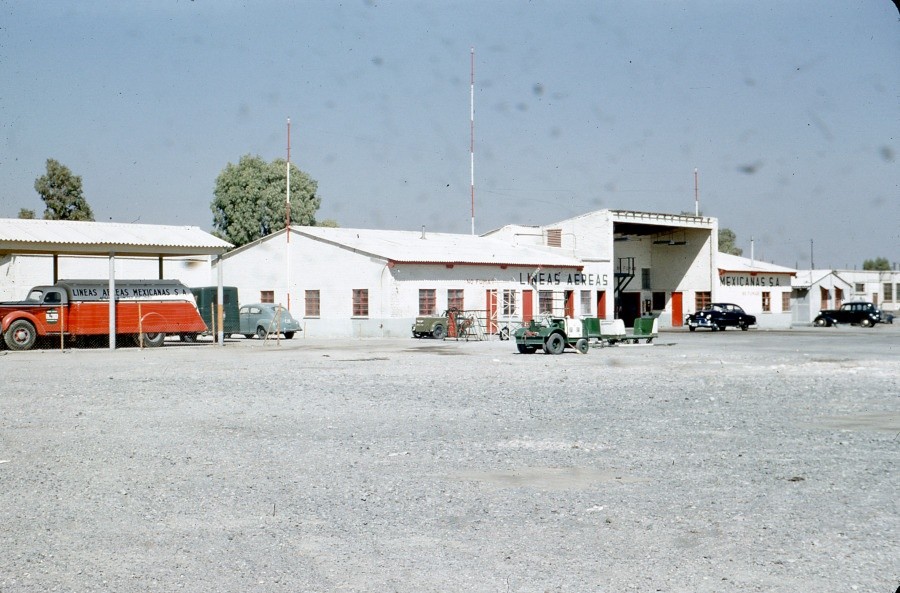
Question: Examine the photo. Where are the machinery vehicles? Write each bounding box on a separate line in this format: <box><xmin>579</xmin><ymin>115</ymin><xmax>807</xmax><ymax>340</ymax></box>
<box><xmin>413</xmin><ymin>307</ymin><xmax>470</xmax><ymax>339</ymax></box>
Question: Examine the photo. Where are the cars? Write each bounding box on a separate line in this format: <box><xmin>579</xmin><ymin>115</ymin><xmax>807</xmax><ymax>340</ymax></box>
<box><xmin>685</xmin><ymin>302</ymin><xmax>758</xmax><ymax>332</ymax></box>
<box><xmin>223</xmin><ymin>304</ymin><xmax>302</xmax><ymax>340</ymax></box>
<box><xmin>814</xmin><ymin>302</ymin><xmax>893</xmax><ymax>328</ymax></box>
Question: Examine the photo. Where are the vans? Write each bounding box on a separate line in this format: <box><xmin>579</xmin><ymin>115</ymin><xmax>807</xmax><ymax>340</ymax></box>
<box><xmin>187</xmin><ymin>286</ymin><xmax>239</xmax><ymax>339</ymax></box>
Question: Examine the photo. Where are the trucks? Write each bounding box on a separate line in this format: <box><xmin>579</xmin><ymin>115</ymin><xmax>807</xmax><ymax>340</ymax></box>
<box><xmin>514</xmin><ymin>310</ymin><xmax>659</xmax><ymax>354</ymax></box>
<box><xmin>0</xmin><ymin>279</ymin><xmax>209</xmax><ymax>347</ymax></box>
